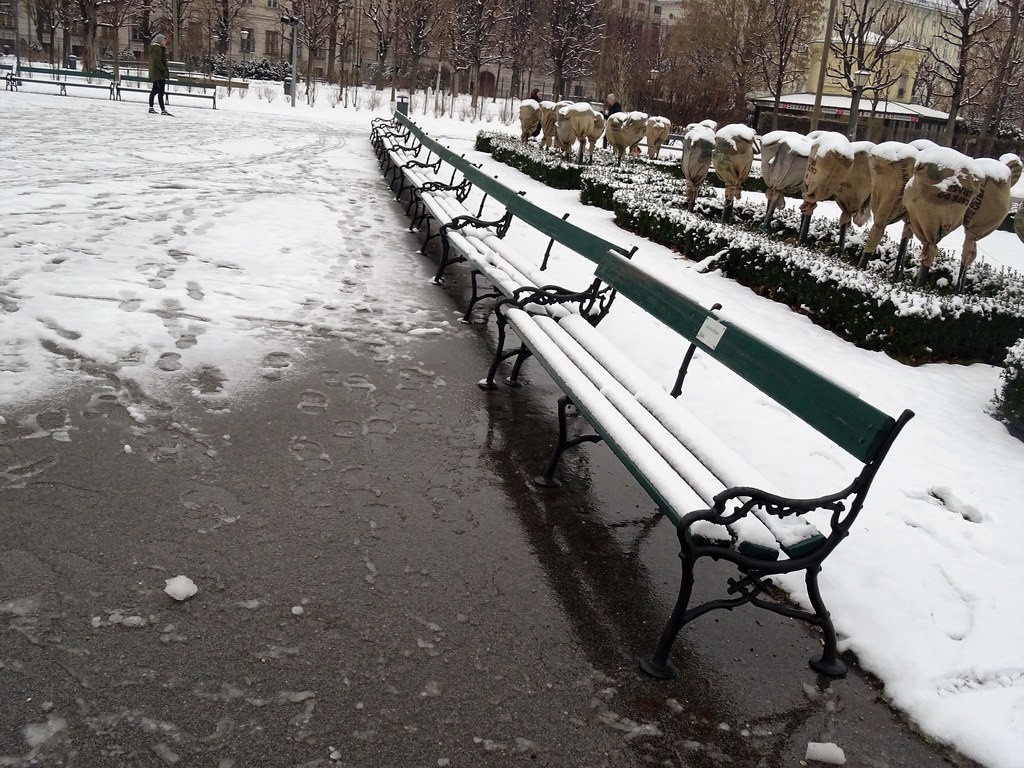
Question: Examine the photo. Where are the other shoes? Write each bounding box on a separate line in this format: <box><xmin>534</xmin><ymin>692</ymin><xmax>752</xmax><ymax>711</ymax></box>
<box><xmin>148</xmin><ymin>107</ymin><xmax>159</xmax><ymax>114</ymax></box>
<box><xmin>161</xmin><ymin>110</ymin><xmax>172</xmax><ymax>115</ymax></box>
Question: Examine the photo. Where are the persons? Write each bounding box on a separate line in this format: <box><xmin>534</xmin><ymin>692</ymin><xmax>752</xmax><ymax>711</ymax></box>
<box><xmin>149</xmin><ymin>34</ymin><xmax>172</xmax><ymax>115</ymax></box>
<box><xmin>529</xmin><ymin>88</ymin><xmax>541</xmax><ymax>136</ymax></box>
<box><xmin>600</xmin><ymin>92</ymin><xmax>623</xmax><ymax>149</ymax></box>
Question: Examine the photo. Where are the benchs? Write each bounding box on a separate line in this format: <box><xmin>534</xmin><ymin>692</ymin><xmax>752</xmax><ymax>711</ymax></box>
<box><xmin>0</xmin><ymin>64</ymin><xmax>217</xmax><ymax>108</ymax></box>
<box><xmin>369</xmin><ymin>109</ymin><xmax>913</xmax><ymax>687</ymax></box>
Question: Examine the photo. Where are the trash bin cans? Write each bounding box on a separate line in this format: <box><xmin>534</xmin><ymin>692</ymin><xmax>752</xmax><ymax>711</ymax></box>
<box><xmin>397</xmin><ymin>95</ymin><xmax>408</xmax><ymax>124</ymax></box>
<box><xmin>284</xmin><ymin>77</ymin><xmax>292</xmax><ymax>95</ymax></box>
<box><xmin>69</xmin><ymin>55</ymin><xmax>76</xmax><ymax>69</ymax></box>
